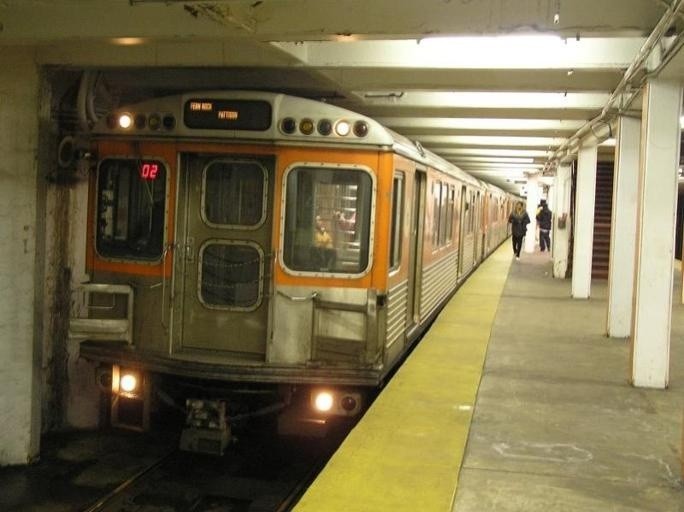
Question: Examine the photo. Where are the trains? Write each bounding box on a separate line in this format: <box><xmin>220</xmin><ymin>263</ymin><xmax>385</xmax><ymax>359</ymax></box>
<box><xmin>65</xmin><ymin>89</ymin><xmax>526</xmax><ymax>473</ymax></box>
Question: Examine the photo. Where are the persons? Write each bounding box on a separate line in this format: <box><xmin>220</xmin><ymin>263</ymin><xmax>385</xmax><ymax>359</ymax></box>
<box><xmin>313</xmin><ymin>209</ymin><xmax>357</xmax><ymax>273</ymax></box>
<box><xmin>535</xmin><ymin>200</ymin><xmax>552</xmax><ymax>252</ymax></box>
<box><xmin>508</xmin><ymin>201</ymin><xmax>530</xmax><ymax>261</ymax></box>
